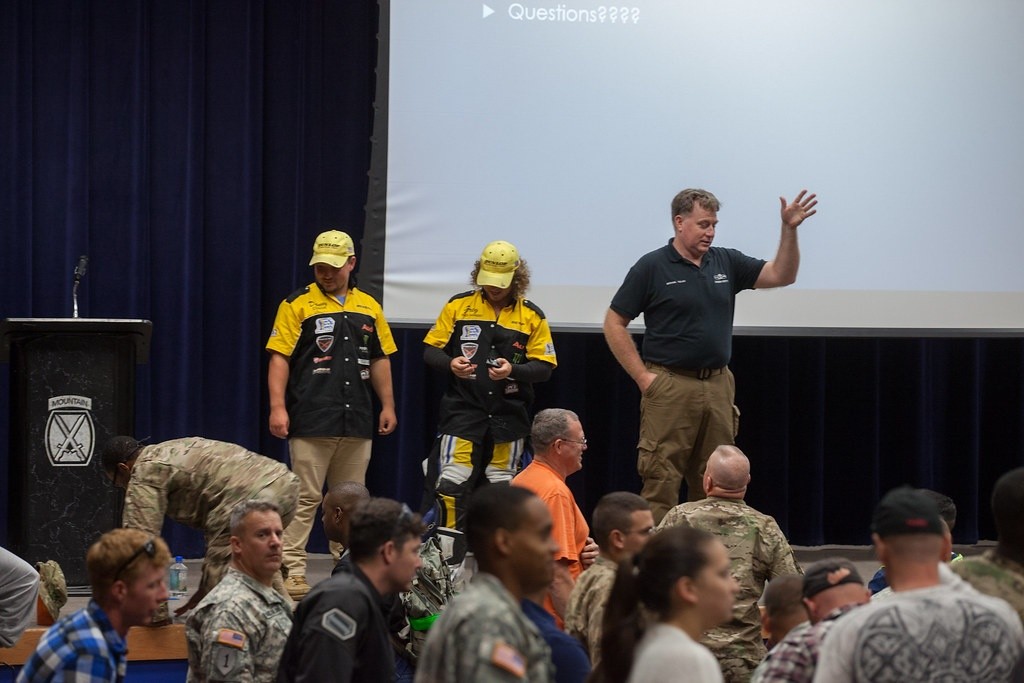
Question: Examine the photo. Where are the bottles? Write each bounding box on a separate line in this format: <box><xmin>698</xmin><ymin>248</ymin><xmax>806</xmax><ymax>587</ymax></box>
<box><xmin>167</xmin><ymin>556</ymin><xmax>188</xmax><ymax>600</ymax></box>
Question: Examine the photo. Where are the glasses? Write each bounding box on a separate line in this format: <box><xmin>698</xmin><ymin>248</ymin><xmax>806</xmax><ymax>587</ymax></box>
<box><xmin>391</xmin><ymin>502</ymin><xmax>414</xmax><ymax>539</ymax></box>
<box><xmin>106</xmin><ymin>471</ymin><xmax>118</xmax><ymax>494</ymax></box>
<box><xmin>117</xmin><ymin>540</ymin><xmax>156</xmax><ymax>578</ymax></box>
<box><xmin>553</xmin><ymin>438</ymin><xmax>588</xmax><ymax>447</ymax></box>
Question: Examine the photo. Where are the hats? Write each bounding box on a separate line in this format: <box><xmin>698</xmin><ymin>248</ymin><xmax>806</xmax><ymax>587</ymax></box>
<box><xmin>37</xmin><ymin>561</ymin><xmax>68</xmax><ymax>622</ymax></box>
<box><xmin>875</xmin><ymin>487</ymin><xmax>941</xmax><ymax>539</ymax></box>
<box><xmin>476</xmin><ymin>241</ymin><xmax>520</xmax><ymax>289</ymax></box>
<box><xmin>802</xmin><ymin>555</ymin><xmax>863</xmax><ymax>598</ymax></box>
<box><xmin>309</xmin><ymin>230</ymin><xmax>355</xmax><ymax>268</ymax></box>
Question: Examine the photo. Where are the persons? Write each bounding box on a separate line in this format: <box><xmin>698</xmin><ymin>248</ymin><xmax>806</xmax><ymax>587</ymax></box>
<box><xmin>265</xmin><ymin>231</ymin><xmax>399</xmax><ymax>601</ymax></box>
<box><xmin>602</xmin><ymin>189</ymin><xmax>819</xmax><ymax>529</ymax></box>
<box><xmin>421</xmin><ymin>241</ymin><xmax>557</xmax><ymax>572</ymax></box>
<box><xmin>0</xmin><ymin>407</ymin><xmax>1024</xmax><ymax>683</ymax></box>
<box><xmin>98</xmin><ymin>432</ymin><xmax>299</xmax><ymax>617</ymax></box>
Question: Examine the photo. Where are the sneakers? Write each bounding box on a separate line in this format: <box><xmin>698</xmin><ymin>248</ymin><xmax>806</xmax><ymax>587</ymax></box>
<box><xmin>283</xmin><ymin>575</ymin><xmax>312</xmax><ymax>600</ymax></box>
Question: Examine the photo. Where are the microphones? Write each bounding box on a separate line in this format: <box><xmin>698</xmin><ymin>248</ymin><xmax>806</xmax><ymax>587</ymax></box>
<box><xmin>75</xmin><ymin>254</ymin><xmax>89</xmax><ymax>280</ymax></box>
